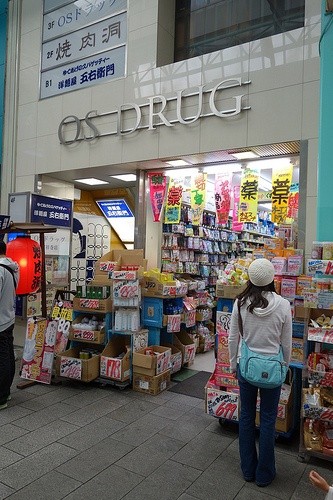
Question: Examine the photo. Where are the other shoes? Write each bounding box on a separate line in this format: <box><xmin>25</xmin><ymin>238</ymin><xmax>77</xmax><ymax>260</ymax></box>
<box><xmin>239</xmin><ymin>459</ymin><xmax>255</xmax><ymax>482</ymax></box>
<box><xmin>255</xmin><ymin>472</ymin><xmax>276</xmax><ymax>487</ymax></box>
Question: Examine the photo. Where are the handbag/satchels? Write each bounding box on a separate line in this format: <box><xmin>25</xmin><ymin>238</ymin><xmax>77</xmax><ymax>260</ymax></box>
<box><xmin>239</xmin><ymin>340</ymin><xmax>289</xmax><ymax>390</ymax></box>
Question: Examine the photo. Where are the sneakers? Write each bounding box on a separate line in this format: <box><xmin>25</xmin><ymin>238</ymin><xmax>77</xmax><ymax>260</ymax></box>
<box><xmin>0</xmin><ymin>402</ymin><xmax>7</xmax><ymax>410</ymax></box>
<box><xmin>7</xmin><ymin>394</ymin><xmax>12</xmax><ymax>400</ymax></box>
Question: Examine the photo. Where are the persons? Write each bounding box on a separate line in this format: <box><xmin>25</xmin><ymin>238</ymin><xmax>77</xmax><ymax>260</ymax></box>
<box><xmin>227</xmin><ymin>257</ymin><xmax>292</xmax><ymax>488</ymax></box>
<box><xmin>0</xmin><ymin>240</ymin><xmax>19</xmax><ymax>409</ymax></box>
<box><xmin>309</xmin><ymin>470</ymin><xmax>332</xmax><ymax>500</ymax></box>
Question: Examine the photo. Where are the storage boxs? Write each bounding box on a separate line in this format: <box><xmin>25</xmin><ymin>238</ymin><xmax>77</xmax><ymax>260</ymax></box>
<box><xmin>215</xmin><ymin>284</ymin><xmax>247</xmax><ymax>299</ymax></box>
<box><xmin>55</xmin><ymin>249</ymin><xmax>213</xmax><ymax>389</ymax></box>
<box><xmin>255</xmin><ymin>397</ymin><xmax>293</xmax><ymax>433</ymax></box>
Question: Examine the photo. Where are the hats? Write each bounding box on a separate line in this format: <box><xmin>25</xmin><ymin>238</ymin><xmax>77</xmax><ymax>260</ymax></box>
<box><xmin>248</xmin><ymin>258</ymin><xmax>275</xmax><ymax>286</ymax></box>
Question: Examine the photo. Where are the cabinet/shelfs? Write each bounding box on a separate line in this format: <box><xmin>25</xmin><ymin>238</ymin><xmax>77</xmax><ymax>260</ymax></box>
<box><xmin>159</xmin><ymin>201</ymin><xmax>333</xmax><ymax>466</ymax></box>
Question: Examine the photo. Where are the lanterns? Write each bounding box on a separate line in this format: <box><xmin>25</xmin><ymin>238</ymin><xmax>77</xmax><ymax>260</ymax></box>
<box><xmin>4</xmin><ymin>235</ymin><xmax>42</xmax><ymax>296</ymax></box>
